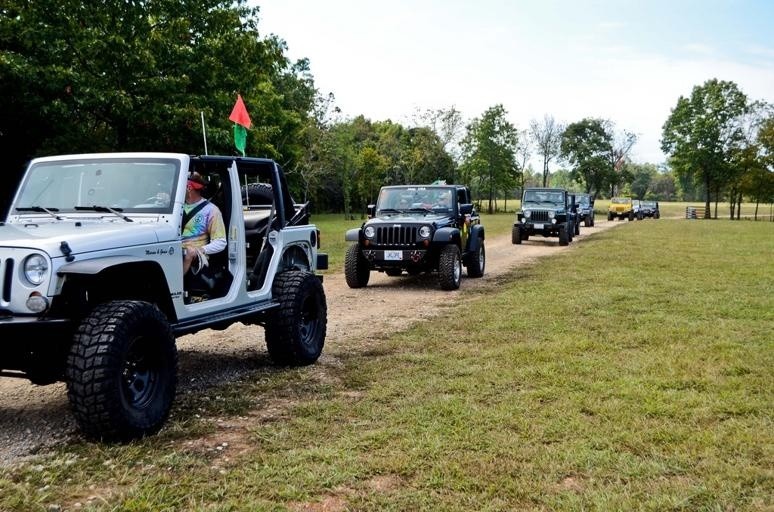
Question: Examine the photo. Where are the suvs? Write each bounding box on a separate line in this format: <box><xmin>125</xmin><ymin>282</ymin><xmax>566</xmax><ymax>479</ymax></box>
<box><xmin>631</xmin><ymin>200</ymin><xmax>643</xmax><ymax>220</ymax></box>
<box><xmin>608</xmin><ymin>197</ymin><xmax>634</xmax><ymax>221</ymax></box>
<box><xmin>511</xmin><ymin>188</ymin><xmax>579</xmax><ymax>246</ymax></box>
<box><xmin>642</xmin><ymin>201</ymin><xmax>660</xmax><ymax>219</ymax></box>
<box><xmin>344</xmin><ymin>184</ymin><xmax>485</xmax><ymax>291</ymax></box>
<box><xmin>568</xmin><ymin>193</ymin><xmax>594</xmax><ymax>227</ymax></box>
<box><xmin>0</xmin><ymin>152</ymin><xmax>329</xmax><ymax>440</ymax></box>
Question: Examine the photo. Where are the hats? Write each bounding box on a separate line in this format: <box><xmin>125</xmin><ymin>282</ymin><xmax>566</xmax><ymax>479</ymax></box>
<box><xmin>187</xmin><ymin>172</ymin><xmax>207</xmax><ymax>192</ymax></box>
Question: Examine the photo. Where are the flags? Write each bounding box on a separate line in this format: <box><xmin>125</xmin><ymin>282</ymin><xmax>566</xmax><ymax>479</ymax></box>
<box><xmin>228</xmin><ymin>95</ymin><xmax>250</xmax><ymax>130</ymax></box>
<box><xmin>233</xmin><ymin>122</ymin><xmax>247</xmax><ymax>154</ymax></box>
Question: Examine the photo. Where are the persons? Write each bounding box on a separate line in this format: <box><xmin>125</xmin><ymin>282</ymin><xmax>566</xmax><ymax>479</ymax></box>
<box><xmin>154</xmin><ymin>175</ymin><xmax>227</xmax><ymax>276</ymax></box>
<box><xmin>397</xmin><ymin>197</ymin><xmax>411</xmax><ymax>210</ymax></box>
<box><xmin>433</xmin><ymin>193</ymin><xmax>472</xmax><ymax>225</ymax></box>
